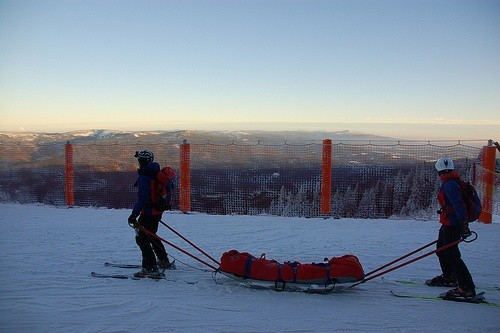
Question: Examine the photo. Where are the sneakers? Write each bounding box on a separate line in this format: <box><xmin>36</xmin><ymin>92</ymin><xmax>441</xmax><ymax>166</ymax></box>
<box><xmin>425</xmin><ymin>274</ymin><xmax>460</xmax><ymax>287</ymax></box>
<box><xmin>437</xmin><ymin>287</ymin><xmax>476</xmax><ymax>300</ymax></box>
<box><xmin>134</xmin><ymin>267</ymin><xmax>159</xmax><ymax>277</ymax></box>
<box><xmin>156</xmin><ymin>259</ymin><xmax>169</xmax><ymax>269</ymax></box>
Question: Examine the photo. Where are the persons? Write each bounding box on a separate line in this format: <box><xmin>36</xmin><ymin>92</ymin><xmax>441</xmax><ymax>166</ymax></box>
<box><xmin>127</xmin><ymin>151</ymin><xmax>169</xmax><ymax>278</ymax></box>
<box><xmin>433</xmin><ymin>157</ymin><xmax>478</xmax><ymax>297</ymax></box>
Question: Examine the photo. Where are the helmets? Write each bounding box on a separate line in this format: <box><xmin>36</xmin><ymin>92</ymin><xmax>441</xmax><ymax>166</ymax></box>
<box><xmin>134</xmin><ymin>150</ymin><xmax>154</xmax><ymax>166</ymax></box>
<box><xmin>434</xmin><ymin>157</ymin><xmax>454</xmax><ymax>176</ymax></box>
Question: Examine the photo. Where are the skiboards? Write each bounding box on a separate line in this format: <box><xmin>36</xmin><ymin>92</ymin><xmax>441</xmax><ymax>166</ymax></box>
<box><xmin>389</xmin><ymin>279</ymin><xmax>500</xmax><ymax>307</ymax></box>
<box><xmin>91</xmin><ymin>262</ymin><xmax>199</xmax><ymax>285</ymax></box>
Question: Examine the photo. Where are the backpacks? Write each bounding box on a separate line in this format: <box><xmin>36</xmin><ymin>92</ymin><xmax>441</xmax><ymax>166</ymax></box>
<box><xmin>441</xmin><ymin>177</ymin><xmax>481</xmax><ymax>221</ymax></box>
<box><xmin>146</xmin><ymin>167</ymin><xmax>176</xmax><ymax>212</ymax></box>
